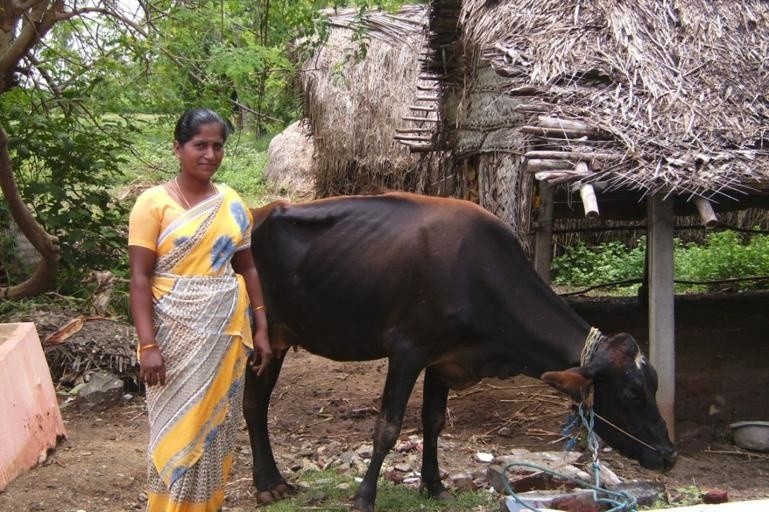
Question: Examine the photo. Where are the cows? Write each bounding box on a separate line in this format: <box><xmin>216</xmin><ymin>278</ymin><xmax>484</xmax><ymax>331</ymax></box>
<box><xmin>231</xmin><ymin>191</ymin><xmax>678</xmax><ymax>512</ymax></box>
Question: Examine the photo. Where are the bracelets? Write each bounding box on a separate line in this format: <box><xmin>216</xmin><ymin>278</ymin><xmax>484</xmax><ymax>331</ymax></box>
<box><xmin>138</xmin><ymin>343</ymin><xmax>161</xmax><ymax>350</ymax></box>
<box><xmin>253</xmin><ymin>304</ymin><xmax>266</xmax><ymax>312</ymax></box>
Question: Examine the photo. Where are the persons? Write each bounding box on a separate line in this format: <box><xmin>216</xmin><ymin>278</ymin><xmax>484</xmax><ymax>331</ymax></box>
<box><xmin>126</xmin><ymin>108</ymin><xmax>273</xmax><ymax>511</ymax></box>
